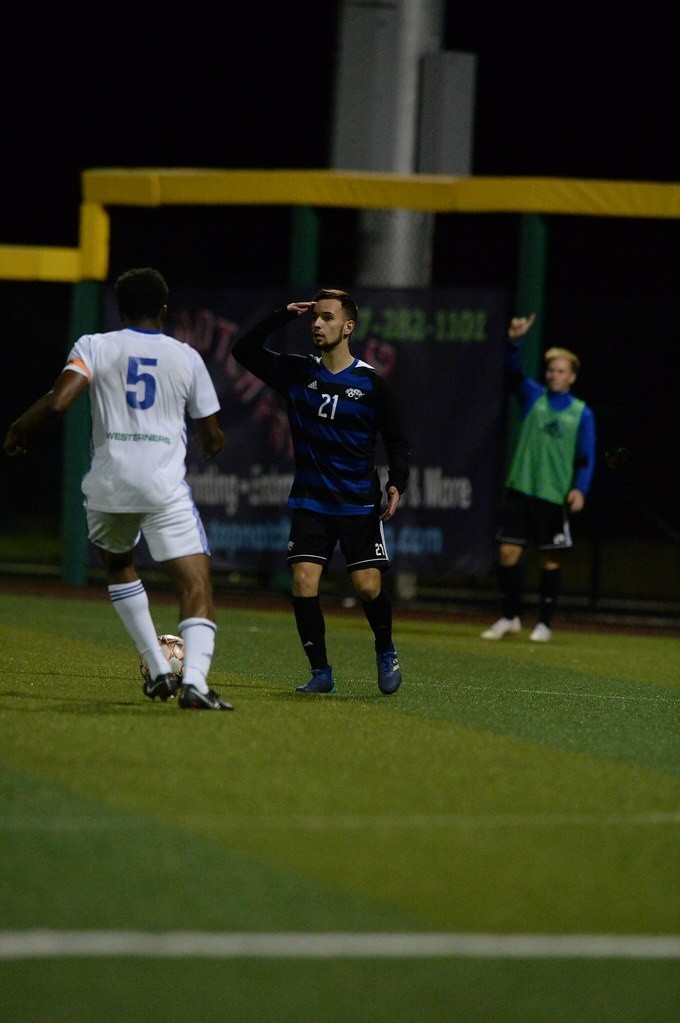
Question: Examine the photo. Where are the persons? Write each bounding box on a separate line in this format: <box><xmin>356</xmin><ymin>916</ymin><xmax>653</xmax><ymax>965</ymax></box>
<box><xmin>230</xmin><ymin>289</ymin><xmax>411</xmax><ymax>695</ymax></box>
<box><xmin>3</xmin><ymin>268</ymin><xmax>234</xmax><ymax>711</ymax></box>
<box><xmin>478</xmin><ymin>310</ymin><xmax>596</xmax><ymax>643</ymax></box>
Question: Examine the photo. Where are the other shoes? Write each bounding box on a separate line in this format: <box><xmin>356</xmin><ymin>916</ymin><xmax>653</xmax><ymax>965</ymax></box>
<box><xmin>528</xmin><ymin>622</ymin><xmax>551</xmax><ymax>642</ymax></box>
<box><xmin>481</xmin><ymin>617</ymin><xmax>523</xmax><ymax>640</ymax></box>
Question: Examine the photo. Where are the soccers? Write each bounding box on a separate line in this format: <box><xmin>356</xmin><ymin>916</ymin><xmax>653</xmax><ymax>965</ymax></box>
<box><xmin>139</xmin><ymin>634</ymin><xmax>185</xmax><ymax>684</ymax></box>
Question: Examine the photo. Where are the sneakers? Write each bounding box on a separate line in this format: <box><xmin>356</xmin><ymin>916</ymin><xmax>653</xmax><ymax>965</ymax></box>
<box><xmin>297</xmin><ymin>666</ymin><xmax>335</xmax><ymax>697</ymax></box>
<box><xmin>178</xmin><ymin>681</ymin><xmax>233</xmax><ymax>711</ymax></box>
<box><xmin>377</xmin><ymin>645</ymin><xmax>402</xmax><ymax>695</ymax></box>
<box><xmin>143</xmin><ymin>674</ymin><xmax>180</xmax><ymax>702</ymax></box>
